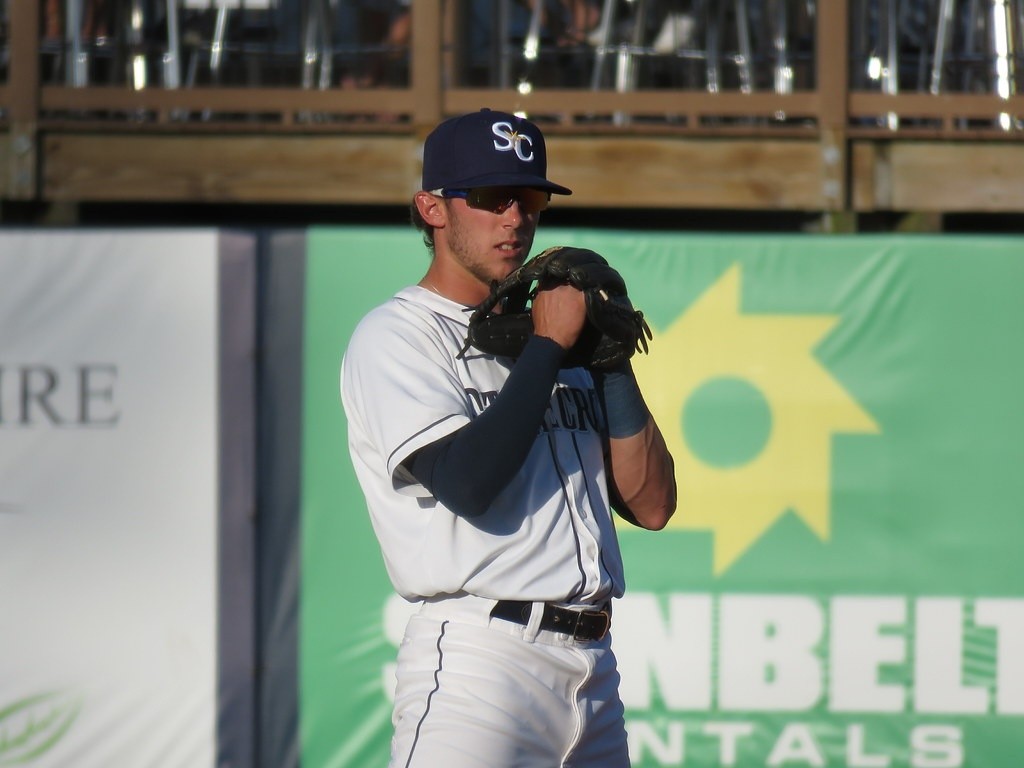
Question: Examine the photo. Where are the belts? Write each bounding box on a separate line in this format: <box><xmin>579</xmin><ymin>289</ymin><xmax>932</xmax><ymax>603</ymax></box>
<box><xmin>490</xmin><ymin>600</ymin><xmax>611</xmax><ymax>642</ymax></box>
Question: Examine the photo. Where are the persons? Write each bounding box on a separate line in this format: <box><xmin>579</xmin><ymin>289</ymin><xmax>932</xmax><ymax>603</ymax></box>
<box><xmin>339</xmin><ymin>108</ymin><xmax>677</xmax><ymax>768</ymax></box>
<box><xmin>269</xmin><ymin>0</ymin><xmax>600</xmax><ymax>90</ymax></box>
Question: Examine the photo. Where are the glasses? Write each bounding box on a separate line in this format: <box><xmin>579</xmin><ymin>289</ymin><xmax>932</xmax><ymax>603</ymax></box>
<box><xmin>430</xmin><ymin>188</ymin><xmax>549</xmax><ymax>213</ymax></box>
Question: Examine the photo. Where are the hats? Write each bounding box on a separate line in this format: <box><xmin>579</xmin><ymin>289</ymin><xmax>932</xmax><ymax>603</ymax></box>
<box><xmin>421</xmin><ymin>107</ymin><xmax>573</xmax><ymax>196</ymax></box>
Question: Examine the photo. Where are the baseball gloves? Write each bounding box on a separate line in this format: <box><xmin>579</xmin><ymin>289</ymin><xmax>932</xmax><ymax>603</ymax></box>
<box><xmin>454</xmin><ymin>242</ymin><xmax>655</xmax><ymax>386</ymax></box>
